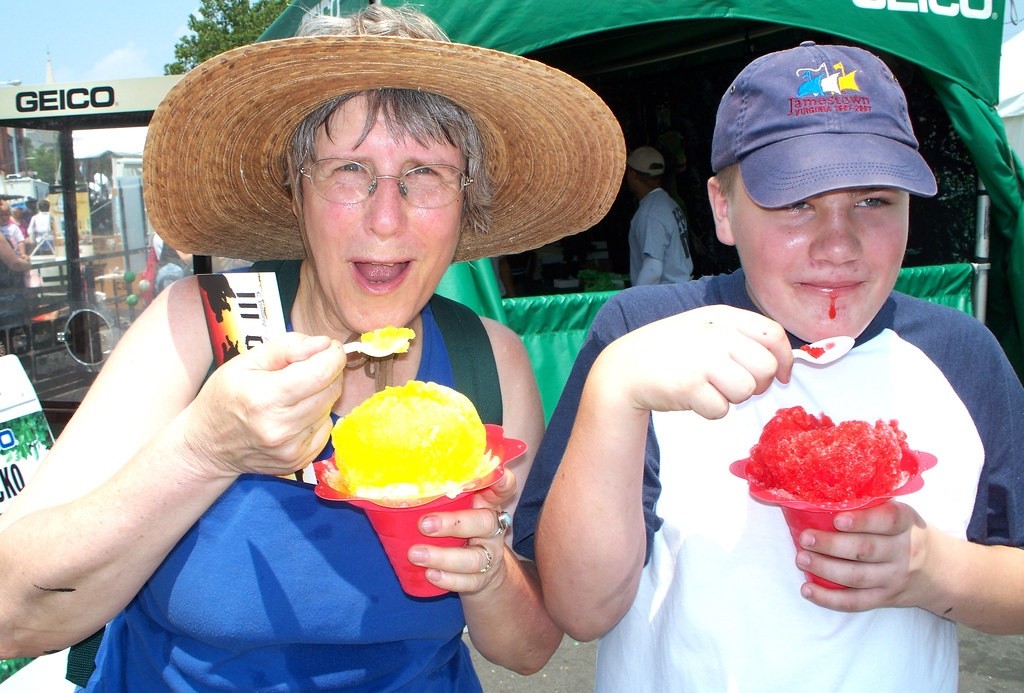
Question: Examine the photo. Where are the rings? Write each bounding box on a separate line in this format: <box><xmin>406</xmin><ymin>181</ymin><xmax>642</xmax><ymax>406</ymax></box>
<box><xmin>490</xmin><ymin>506</ymin><xmax>513</xmax><ymax>537</ymax></box>
<box><xmin>469</xmin><ymin>543</ymin><xmax>493</xmax><ymax>574</ymax></box>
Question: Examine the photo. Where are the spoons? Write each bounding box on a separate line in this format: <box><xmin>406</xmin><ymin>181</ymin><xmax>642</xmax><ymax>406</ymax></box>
<box><xmin>794</xmin><ymin>335</ymin><xmax>855</xmax><ymax>365</ymax></box>
<box><xmin>341</xmin><ymin>327</ymin><xmax>411</xmax><ymax>360</ymax></box>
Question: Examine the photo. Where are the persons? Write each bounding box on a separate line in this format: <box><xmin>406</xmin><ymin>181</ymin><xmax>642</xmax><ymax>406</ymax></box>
<box><xmin>622</xmin><ymin>143</ymin><xmax>695</xmax><ymax>289</ymax></box>
<box><xmin>152</xmin><ymin>225</ymin><xmax>225</xmax><ymax>298</ymax></box>
<box><xmin>510</xmin><ymin>38</ymin><xmax>1024</xmax><ymax>693</ymax></box>
<box><xmin>1</xmin><ymin>199</ymin><xmax>32</xmax><ymax>293</ymax></box>
<box><xmin>27</xmin><ymin>200</ymin><xmax>62</xmax><ymax>255</ymax></box>
<box><xmin>0</xmin><ymin>6</ymin><xmax>567</xmax><ymax>693</ymax></box>
<box><xmin>667</xmin><ymin>151</ymin><xmax>695</xmax><ymax>229</ymax></box>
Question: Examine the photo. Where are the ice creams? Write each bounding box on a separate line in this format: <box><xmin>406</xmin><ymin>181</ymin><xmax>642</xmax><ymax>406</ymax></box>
<box><xmin>360</xmin><ymin>326</ymin><xmax>415</xmax><ymax>355</ymax></box>
<box><xmin>330</xmin><ymin>379</ymin><xmax>498</xmax><ymax>507</ymax></box>
<box><xmin>747</xmin><ymin>404</ymin><xmax>920</xmax><ymax>507</ymax></box>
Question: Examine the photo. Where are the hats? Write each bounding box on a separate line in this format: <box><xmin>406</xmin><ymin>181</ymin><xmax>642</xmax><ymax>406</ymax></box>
<box><xmin>142</xmin><ymin>34</ymin><xmax>627</xmax><ymax>262</ymax></box>
<box><xmin>710</xmin><ymin>40</ymin><xmax>938</xmax><ymax>209</ymax></box>
<box><xmin>628</xmin><ymin>147</ymin><xmax>664</xmax><ymax>175</ymax></box>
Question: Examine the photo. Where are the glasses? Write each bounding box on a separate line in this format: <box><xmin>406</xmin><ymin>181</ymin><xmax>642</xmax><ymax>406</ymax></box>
<box><xmin>299</xmin><ymin>157</ymin><xmax>474</xmax><ymax>208</ymax></box>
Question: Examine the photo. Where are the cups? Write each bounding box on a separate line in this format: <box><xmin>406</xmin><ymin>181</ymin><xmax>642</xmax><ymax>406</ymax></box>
<box><xmin>311</xmin><ymin>425</ymin><xmax>527</xmax><ymax>596</ymax></box>
<box><xmin>729</xmin><ymin>451</ymin><xmax>939</xmax><ymax>591</ymax></box>
